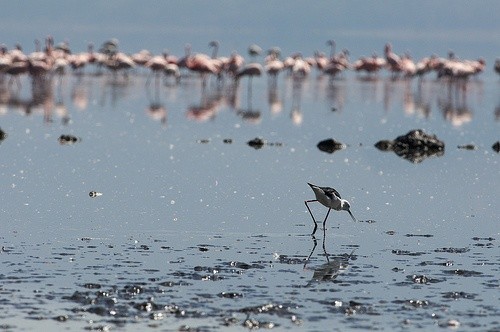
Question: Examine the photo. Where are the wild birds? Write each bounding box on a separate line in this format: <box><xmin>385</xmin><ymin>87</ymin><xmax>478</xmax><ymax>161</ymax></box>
<box><xmin>0</xmin><ymin>33</ymin><xmax>500</xmax><ymax>75</ymax></box>
<box><xmin>0</xmin><ymin>75</ymin><xmax>500</xmax><ymax>129</ymax></box>
<box><xmin>304</xmin><ymin>180</ymin><xmax>358</xmax><ymax>238</ymax></box>
<box><xmin>302</xmin><ymin>238</ymin><xmax>357</xmax><ymax>289</ymax></box>
